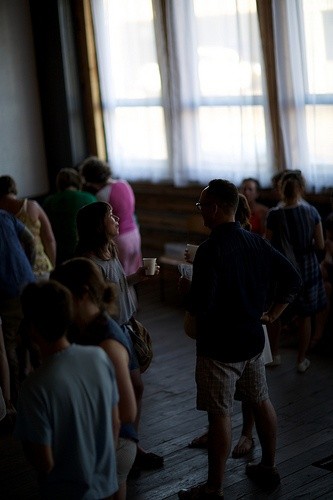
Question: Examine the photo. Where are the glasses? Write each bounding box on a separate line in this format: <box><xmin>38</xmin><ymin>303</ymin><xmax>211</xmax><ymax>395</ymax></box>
<box><xmin>195</xmin><ymin>203</ymin><xmax>214</xmax><ymax>209</ymax></box>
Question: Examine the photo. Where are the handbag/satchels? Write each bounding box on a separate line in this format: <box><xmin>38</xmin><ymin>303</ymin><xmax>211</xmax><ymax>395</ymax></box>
<box><xmin>127</xmin><ymin>316</ymin><xmax>153</xmax><ymax>375</ymax></box>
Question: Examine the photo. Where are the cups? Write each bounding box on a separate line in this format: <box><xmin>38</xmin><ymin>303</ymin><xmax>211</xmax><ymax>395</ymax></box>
<box><xmin>187</xmin><ymin>244</ymin><xmax>199</xmax><ymax>261</ymax></box>
<box><xmin>143</xmin><ymin>258</ymin><xmax>156</xmax><ymax>275</ymax></box>
<box><xmin>178</xmin><ymin>263</ymin><xmax>192</xmax><ymax>282</ymax></box>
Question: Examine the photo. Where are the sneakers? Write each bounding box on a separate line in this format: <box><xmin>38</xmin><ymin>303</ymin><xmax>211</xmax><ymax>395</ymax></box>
<box><xmin>177</xmin><ymin>482</ymin><xmax>225</xmax><ymax>495</ymax></box>
<box><xmin>249</xmin><ymin>464</ymin><xmax>281</xmax><ymax>487</ymax></box>
<box><xmin>265</xmin><ymin>355</ymin><xmax>284</xmax><ymax>364</ymax></box>
<box><xmin>296</xmin><ymin>356</ymin><xmax>307</xmax><ymax>372</ymax></box>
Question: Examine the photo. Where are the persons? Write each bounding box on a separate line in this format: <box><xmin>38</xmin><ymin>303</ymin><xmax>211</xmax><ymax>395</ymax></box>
<box><xmin>184</xmin><ymin>193</ymin><xmax>274</xmax><ymax>459</ymax></box>
<box><xmin>0</xmin><ymin>316</ymin><xmax>17</xmax><ymax>421</ymax></box>
<box><xmin>78</xmin><ymin>157</ymin><xmax>143</xmax><ymax>276</ymax></box>
<box><xmin>49</xmin><ymin>257</ymin><xmax>139</xmax><ymax>500</ymax></box>
<box><xmin>264</xmin><ymin>172</ymin><xmax>328</xmax><ymax>373</ymax></box>
<box><xmin>0</xmin><ymin>176</ymin><xmax>57</xmax><ymax>281</ymax></box>
<box><xmin>42</xmin><ymin>168</ymin><xmax>98</xmax><ymax>266</ymax></box>
<box><xmin>240</xmin><ymin>178</ymin><xmax>269</xmax><ymax>238</ymax></box>
<box><xmin>0</xmin><ymin>209</ymin><xmax>40</xmax><ymax>411</ymax></box>
<box><xmin>177</xmin><ymin>179</ymin><xmax>303</xmax><ymax>500</ymax></box>
<box><xmin>308</xmin><ymin>213</ymin><xmax>333</xmax><ymax>348</ymax></box>
<box><xmin>74</xmin><ymin>201</ymin><xmax>164</xmax><ymax>481</ymax></box>
<box><xmin>272</xmin><ymin>170</ymin><xmax>310</xmax><ymax>207</ymax></box>
<box><xmin>13</xmin><ymin>280</ymin><xmax>121</xmax><ymax>500</ymax></box>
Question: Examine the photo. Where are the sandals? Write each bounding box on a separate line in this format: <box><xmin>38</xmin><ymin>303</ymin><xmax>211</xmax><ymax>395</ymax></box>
<box><xmin>230</xmin><ymin>432</ymin><xmax>256</xmax><ymax>455</ymax></box>
<box><xmin>186</xmin><ymin>430</ymin><xmax>208</xmax><ymax>449</ymax></box>
